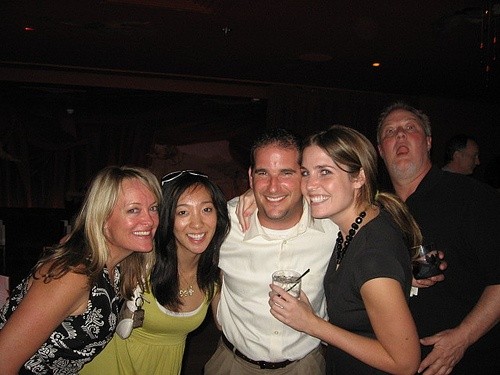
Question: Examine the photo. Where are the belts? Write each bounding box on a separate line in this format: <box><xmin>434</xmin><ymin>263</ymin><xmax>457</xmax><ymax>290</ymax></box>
<box><xmin>221</xmin><ymin>331</ymin><xmax>297</xmax><ymax>369</ymax></box>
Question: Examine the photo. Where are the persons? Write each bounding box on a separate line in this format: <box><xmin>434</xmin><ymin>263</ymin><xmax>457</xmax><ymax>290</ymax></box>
<box><xmin>0</xmin><ymin>165</ymin><xmax>163</xmax><ymax>375</ymax></box>
<box><xmin>439</xmin><ymin>133</ymin><xmax>481</xmax><ymax>176</ymax></box>
<box><xmin>369</xmin><ymin>102</ymin><xmax>500</xmax><ymax>375</ymax></box>
<box><xmin>268</xmin><ymin>123</ymin><xmax>429</xmax><ymax>375</ymax></box>
<box><xmin>71</xmin><ymin>170</ymin><xmax>231</xmax><ymax>375</ymax></box>
<box><xmin>203</xmin><ymin>128</ymin><xmax>447</xmax><ymax>375</ymax></box>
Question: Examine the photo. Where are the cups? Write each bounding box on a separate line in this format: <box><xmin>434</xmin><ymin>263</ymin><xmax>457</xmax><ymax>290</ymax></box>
<box><xmin>272</xmin><ymin>270</ymin><xmax>301</xmax><ymax>304</ymax></box>
<box><xmin>408</xmin><ymin>238</ymin><xmax>444</xmax><ymax>280</ymax></box>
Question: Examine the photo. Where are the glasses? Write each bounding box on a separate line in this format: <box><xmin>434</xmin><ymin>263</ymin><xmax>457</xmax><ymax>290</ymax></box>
<box><xmin>161</xmin><ymin>170</ymin><xmax>209</xmax><ymax>189</ymax></box>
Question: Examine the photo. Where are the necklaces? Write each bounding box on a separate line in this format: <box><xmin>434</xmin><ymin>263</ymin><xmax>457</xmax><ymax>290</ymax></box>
<box><xmin>179</xmin><ymin>276</ymin><xmax>188</xmax><ymax>299</ymax></box>
<box><xmin>336</xmin><ymin>204</ymin><xmax>372</xmax><ymax>270</ymax></box>
<box><xmin>179</xmin><ymin>264</ymin><xmax>197</xmax><ymax>296</ymax></box>
